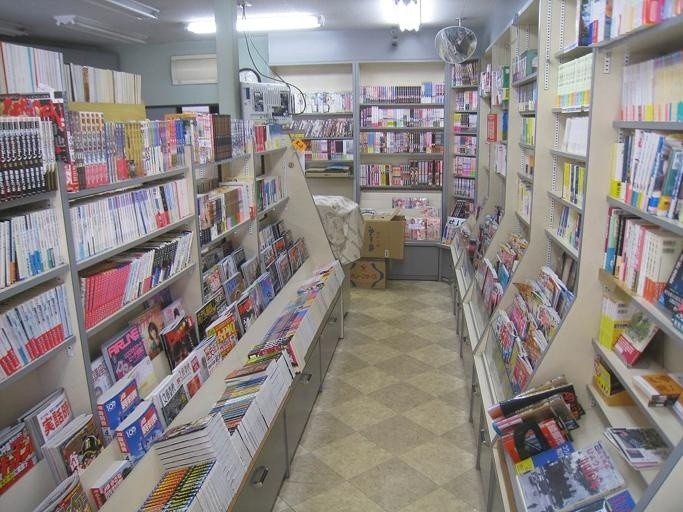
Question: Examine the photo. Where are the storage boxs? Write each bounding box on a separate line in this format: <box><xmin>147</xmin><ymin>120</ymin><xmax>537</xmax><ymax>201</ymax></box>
<box><xmin>350</xmin><ymin>260</ymin><xmax>390</xmax><ymax>289</ymax></box>
<box><xmin>361</xmin><ymin>208</ymin><xmax>406</xmax><ymax>259</ymax></box>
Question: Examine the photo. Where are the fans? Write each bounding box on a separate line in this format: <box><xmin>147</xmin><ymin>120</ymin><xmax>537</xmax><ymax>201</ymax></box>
<box><xmin>435</xmin><ymin>18</ymin><xmax>477</xmax><ymax>64</ymax></box>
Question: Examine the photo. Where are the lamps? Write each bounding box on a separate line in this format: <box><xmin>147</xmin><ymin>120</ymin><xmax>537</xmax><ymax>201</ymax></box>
<box><xmin>398</xmin><ymin>1</ymin><xmax>422</xmax><ymax>32</ymax></box>
<box><xmin>54</xmin><ymin>16</ymin><xmax>147</xmax><ymax>48</ymax></box>
<box><xmin>83</xmin><ymin>0</ymin><xmax>160</xmax><ymax>21</ymax></box>
<box><xmin>186</xmin><ymin>14</ymin><xmax>326</xmax><ymax>34</ymax></box>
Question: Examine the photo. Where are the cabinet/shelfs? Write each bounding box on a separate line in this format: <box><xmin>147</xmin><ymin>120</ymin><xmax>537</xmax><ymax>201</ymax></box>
<box><xmin>0</xmin><ymin>134</ymin><xmax>344</xmax><ymax>512</ymax></box>
<box><xmin>451</xmin><ymin>3</ymin><xmax>683</xmax><ymax>511</ymax></box>
<box><xmin>272</xmin><ymin>60</ymin><xmax>356</xmax><ymax>178</ymax></box>
<box><xmin>446</xmin><ymin>86</ymin><xmax>476</xmax><ymax>244</ymax></box>
<box><xmin>358</xmin><ymin>61</ymin><xmax>444</xmax><ymax>243</ymax></box>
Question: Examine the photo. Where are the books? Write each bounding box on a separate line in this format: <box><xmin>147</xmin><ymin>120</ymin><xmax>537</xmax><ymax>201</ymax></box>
<box><xmin>138</xmin><ymin>111</ymin><xmax>345</xmax><ymax>510</ymax></box>
<box><xmin>344</xmin><ymin>82</ymin><xmax>451</xmax><ymax>243</ymax></box>
<box><xmin>0</xmin><ymin>39</ymin><xmax>142</xmax><ymax>512</ymax></box>
<box><xmin>471</xmin><ymin>51</ymin><xmax>683</xmax><ymax>510</ymax></box>
<box><xmin>579</xmin><ymin>2</ymin><xmax>683</xmax><ymax>49</ymax></box>
<box><xmin>242</xmin><ymin>83</ymin><xmax>346</xmax><ymax>114</ymax></box>
<box><xmin>450</xmin><ymin>60</ymin><xmax>480</xmax><ymax>242</ymax></box>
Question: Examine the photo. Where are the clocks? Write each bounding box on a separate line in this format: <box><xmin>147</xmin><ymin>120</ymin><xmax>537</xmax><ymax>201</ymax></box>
<box><xmin>239</xmin><ymin>68</ymin><xmax>261</xmax><ymax>84</ymax></box>
